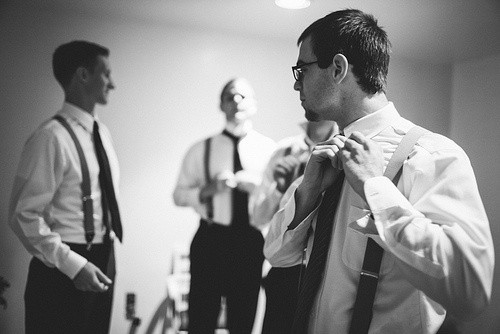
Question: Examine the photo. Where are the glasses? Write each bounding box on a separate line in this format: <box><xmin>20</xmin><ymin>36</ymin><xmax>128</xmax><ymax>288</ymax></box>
<box><xmin>292</xmin><ymin>61</ymin><xmax>322</xmax><ymax>81</ymax></box>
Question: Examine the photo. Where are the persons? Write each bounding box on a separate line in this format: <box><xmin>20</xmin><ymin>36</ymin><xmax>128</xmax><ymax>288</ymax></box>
<box><xmin>262</xmin><ymin>8</ymin><xmax>495</xmax><ymax>334</ymax></box>
<box><xmin>8</xmin><ymin>40</ymin><xmax>123</xmax><ymax>334</ymax></box>
<box><xmin>173</xmin><ymin>77</ymin><xmax>274</xmax><ymax>334</ymax></box>
<box><xmin>255</xmin><ymin>120</ymin><xmax>339</xmax><ymax>333</ymax></box>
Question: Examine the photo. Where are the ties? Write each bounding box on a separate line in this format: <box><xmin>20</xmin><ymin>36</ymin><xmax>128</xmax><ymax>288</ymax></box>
<box><xmin>290</xmin><ymin>133</ymin><xmax>344</xmax><ymax>334</ymax></box>
<box><xmin>93</xmin><ymin>122</ymin><xmax>124</xmax><ymax>244</ymax></box>
<box><xmin>222</xmin><ymin>130</ymin><xmax>250</xmax><ymax>243</ymax></box>
